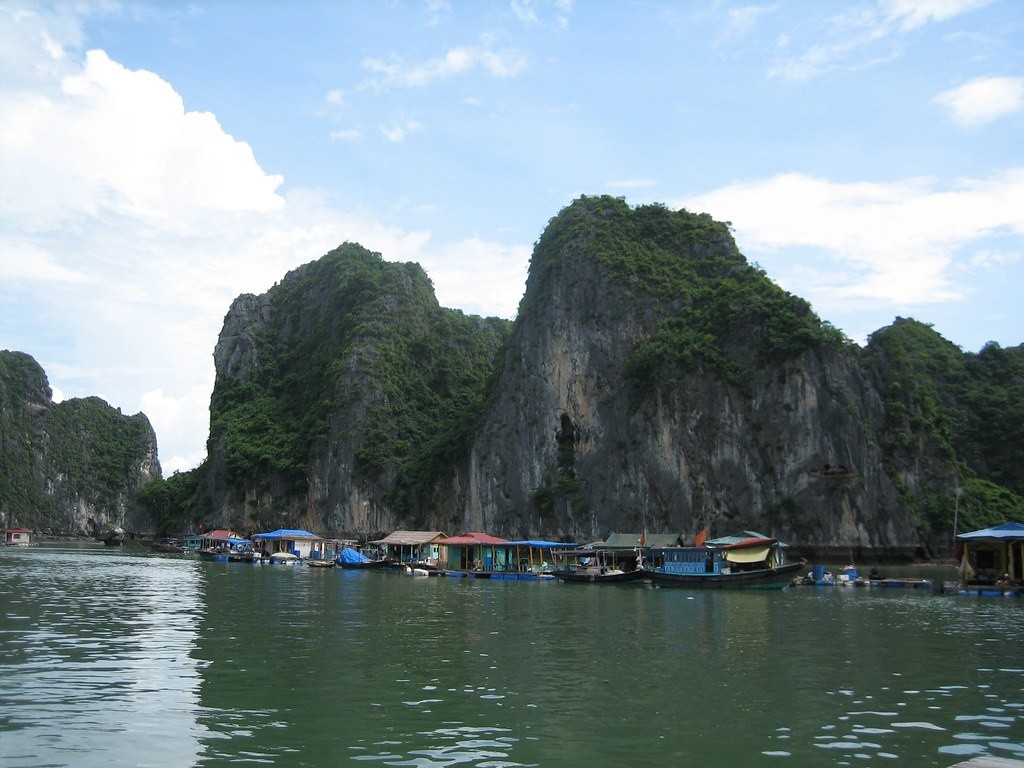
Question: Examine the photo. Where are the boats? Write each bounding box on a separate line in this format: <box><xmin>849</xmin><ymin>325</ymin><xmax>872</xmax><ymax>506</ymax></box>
<box><xmin>801</xmin><ymin>568</ymin><xmax>932</xmax><ymax>588</ymax></box>
<box><xmin>548</xmin><ymin>550</ymin><xmax>641</xmax><ymax>584</ymax></box>
<box><xmin>333</xmin><ymin>548</ymin><xmax>393</xmax><ymax>569</ymax></box>
<box><xmin>640</xmin><ymin>527</ymin><xmax>808</xmax><ymax>590</ymax></box>
<box><xmin>306</xmin><ymin>560</ymin><xmax>336</xmax><ymax>568</ymax></box>
<box><xmin>943</xmin><ymin>521</ymin><xmax>1024</xmax><ymax>597</ymax></box>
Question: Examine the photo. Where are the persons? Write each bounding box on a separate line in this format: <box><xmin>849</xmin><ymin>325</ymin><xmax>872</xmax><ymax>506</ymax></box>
<box><xmin>677</xmin><ymin>534</ymin><xmax>686</xmax><ymax>548</ymax></box>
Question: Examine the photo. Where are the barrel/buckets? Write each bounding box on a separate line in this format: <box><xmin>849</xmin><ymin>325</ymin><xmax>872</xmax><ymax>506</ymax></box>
<box><xmin>311</xmin><ymin>551</ymin><xmax>319</xmax><ymax>559</ymax></box>
<box><xmin>484</xmin><ymin>556</ymin><xmax>493</xmax><ymax>572</ymax></box>
<box><xmin>294</xmin><ymin>550</ymin><xmax>300</xmax><ymax>558</ymax></box>
<box><xmin>813</xmin><ymin>564</ymin><xmax>825</xmax><ymax>580</ymax></box>
